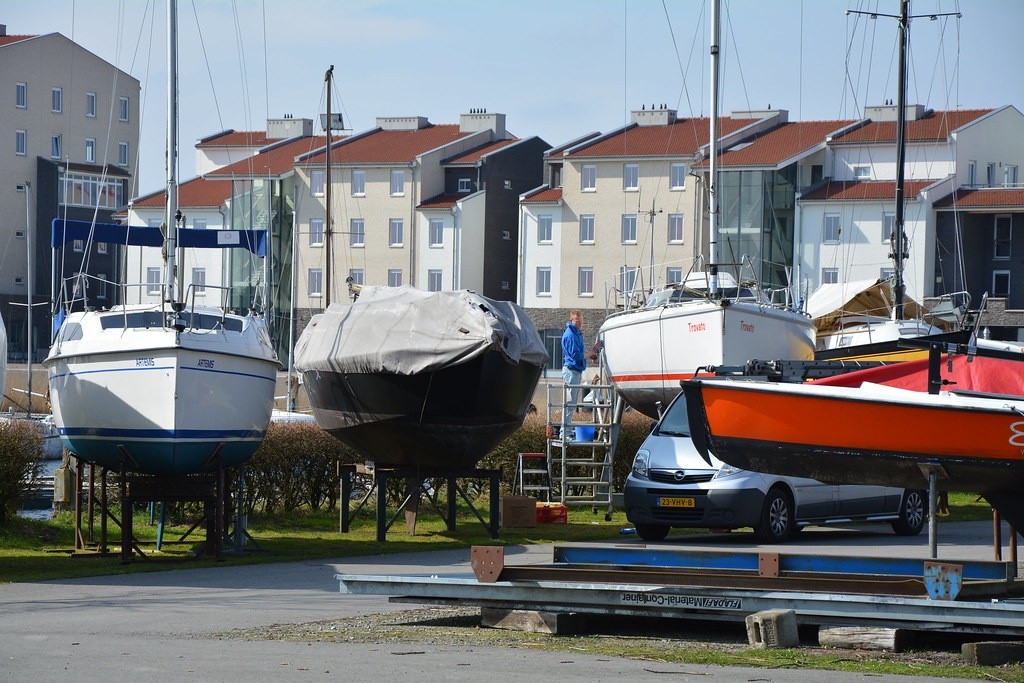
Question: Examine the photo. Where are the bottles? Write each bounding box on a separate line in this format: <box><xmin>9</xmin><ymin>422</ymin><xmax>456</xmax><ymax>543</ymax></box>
<box><xmin>621</xmin><ymin>527</ymin><xmax>636</xmax><ymax>534</ymax></box>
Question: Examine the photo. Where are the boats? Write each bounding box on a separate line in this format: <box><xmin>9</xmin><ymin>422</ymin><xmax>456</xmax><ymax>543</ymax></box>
<box><xmin>681</xmin><ymin>1</ymin><xmax>1024</xmax><ymax>579</ymax></box>
<box><xmin>0</xmin><ymin>1</ymin><xmax>973</xmax><ymax>548</ymax></box>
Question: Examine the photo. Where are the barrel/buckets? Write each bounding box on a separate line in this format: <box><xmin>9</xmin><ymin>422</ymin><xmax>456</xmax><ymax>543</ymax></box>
<box><xmin>574</xmin><ymin>426</ymin><xmax>595</xmax><ymax>442</ymax></box>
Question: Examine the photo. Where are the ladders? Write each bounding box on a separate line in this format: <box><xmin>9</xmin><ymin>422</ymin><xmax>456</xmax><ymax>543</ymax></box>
<box><xmin>511</xmin><ymin>453</ymin><xmax>549</xmax><ymax>504</ymax></box>
<box><xmin>546</xmin><ymin>382</ymin><xmax>614</xmax><ymax>521</ymax></box>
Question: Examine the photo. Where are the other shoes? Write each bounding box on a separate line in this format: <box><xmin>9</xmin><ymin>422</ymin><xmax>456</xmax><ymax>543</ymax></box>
<box><xmin>559</xmin><ymin>433</ymin><xmax>573</xmax><ymax>442</ymax></box>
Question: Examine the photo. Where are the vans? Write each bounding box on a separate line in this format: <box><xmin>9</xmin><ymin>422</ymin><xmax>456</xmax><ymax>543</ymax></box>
<box><xmin>623</xmin><ymin>383</ymin><xmax>928</xmax><ymax>544</ymax></box>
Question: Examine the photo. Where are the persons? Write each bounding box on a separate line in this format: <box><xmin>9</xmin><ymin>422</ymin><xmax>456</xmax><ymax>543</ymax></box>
<box><xmin>559</xmin><ymin>311</ymin><xmax>602</xmax><ymax>442</ymax></box>
